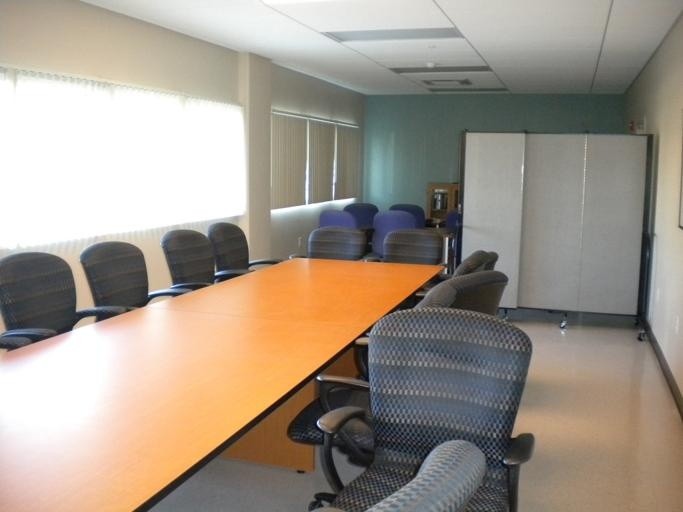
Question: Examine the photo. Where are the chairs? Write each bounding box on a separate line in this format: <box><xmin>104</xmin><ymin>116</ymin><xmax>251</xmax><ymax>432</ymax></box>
<box><xmin>321</xmin><ymin>209</ymin><xmax>363</xmax><ymax>228</ymax></box>
<box><xmin>367</xmin><ymin>229</ymin><xmax>446</xmax><ymax>299</ymax></box>
<box><xmin>488</xmin><ymin>252</ymin><xmax>498</xmax><ymax>271</ymax></box>
<box><xmin>80</xmin><ymin>241</ymin><xmax>190</xmax><ymax>310</ymax></box>
<box><xmin>424</xmin><ymin>249</ymin><xmax>488</xmax><ymax>289</ymax></box>
<box><xmin>372</xmin><ymin>210</ymin><xmax>419</xmax><ymax>257</ymax></box>
<box><xmin>209</xmin><ymin>222</ymin><xmax>281</xmax><ymax>275</ymax></box>
<box><xmin>161</xmin><ymin>229</ymin><xmax>250</xmax><ymax>287</ymax></box>
<box><xmin>343</xmin><ymin>204</ymin><xmax>368</xmax><ymax>235</ymax></box>
<box><xmin>0</xmin><ymin>252</ymin><xmax>130</xmax><ymax>348</ymax></box>
<box><xmin>307</xmin><ymin>308</ymin><xmax>534</xmax><ymax>512</ymax></box>
<box><xmin>289</xmin><ymin>227</ymin><xmax>367</xmax><ymax>260</ymax></box>
<box><xmin>287</xmin><ymin>271</ymin><xmax>512</xmax><ymax>466</ymax></box>
<box><xmin>389</xmin><ymin>204</ymin><xmax>427</xmax><ymax>228</ymax></box>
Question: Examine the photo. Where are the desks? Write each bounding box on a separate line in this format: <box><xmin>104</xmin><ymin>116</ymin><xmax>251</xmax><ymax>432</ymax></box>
<box><xmin>0</xmin><ymin>258</ymin><xmax>444</xmax><ymax>512</ymax></box>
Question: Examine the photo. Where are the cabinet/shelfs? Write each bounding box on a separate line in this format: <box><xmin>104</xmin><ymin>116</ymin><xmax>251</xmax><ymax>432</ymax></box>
<box><xmin>425</xmin><ymin>182</ymin><xmax>457</xmax><ymax>229</ymax></box>
